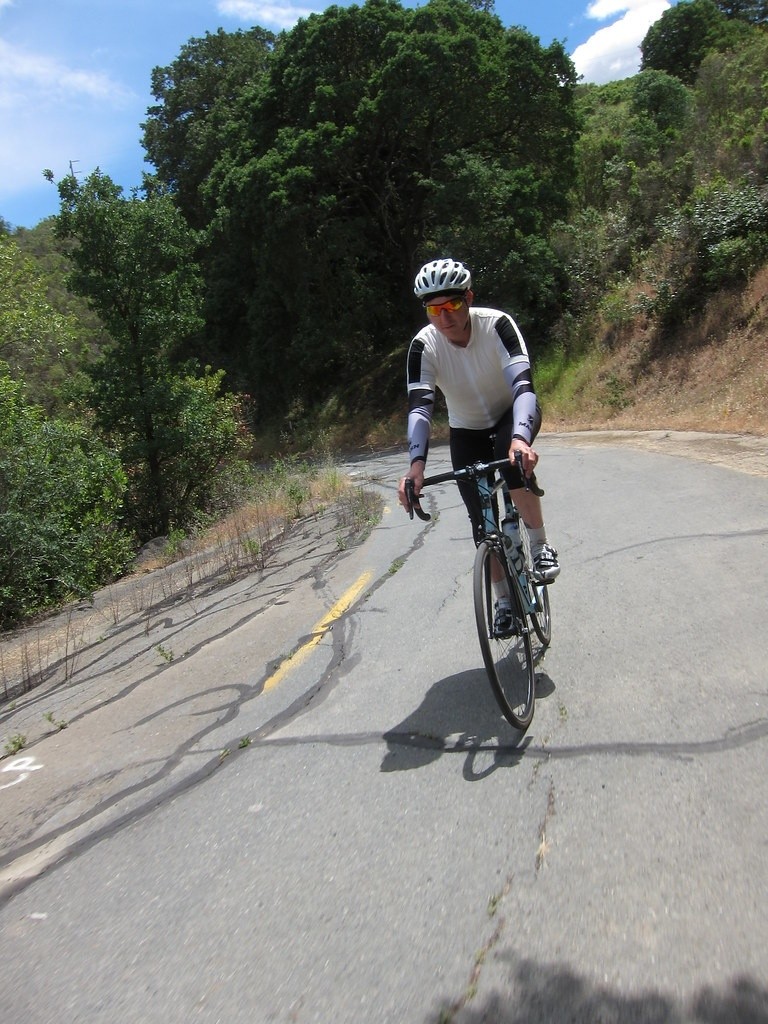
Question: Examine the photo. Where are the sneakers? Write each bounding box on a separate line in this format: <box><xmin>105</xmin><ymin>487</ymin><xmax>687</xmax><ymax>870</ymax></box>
<box><xmin>494</xmin><ymin>602</ymin><xmax>514</xmax><ymax>634</ymax></box>
<box><xmin>530</xmin><ymin>541</ymin><xmax>561</xmax><ymax>583</ymax></box>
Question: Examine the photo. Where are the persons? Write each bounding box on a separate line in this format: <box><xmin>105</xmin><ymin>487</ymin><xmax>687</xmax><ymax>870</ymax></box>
<box><xmin>399</xmin><ymin>259</ymin><xmax>561</xmax><ymax>640</ymax></box>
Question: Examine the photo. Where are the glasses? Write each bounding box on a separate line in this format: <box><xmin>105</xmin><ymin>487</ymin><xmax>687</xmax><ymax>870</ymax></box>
<box><xmin>422</xmin><ymin>295</ymin><xmax>465</xmax><ymax>316</ymax></box>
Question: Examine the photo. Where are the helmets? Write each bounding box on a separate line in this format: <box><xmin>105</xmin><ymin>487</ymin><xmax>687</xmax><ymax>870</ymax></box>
<box><xmin>414</xmin><ymin>258</ymin><xmax>472</xmax><ymax>301</ymax></box>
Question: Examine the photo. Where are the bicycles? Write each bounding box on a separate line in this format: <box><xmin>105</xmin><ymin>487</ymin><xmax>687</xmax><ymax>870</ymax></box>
<box><xmin>404</xmin><ymin>450</ymin><xmax>555</xmax><ymax>730</ymax></box>
